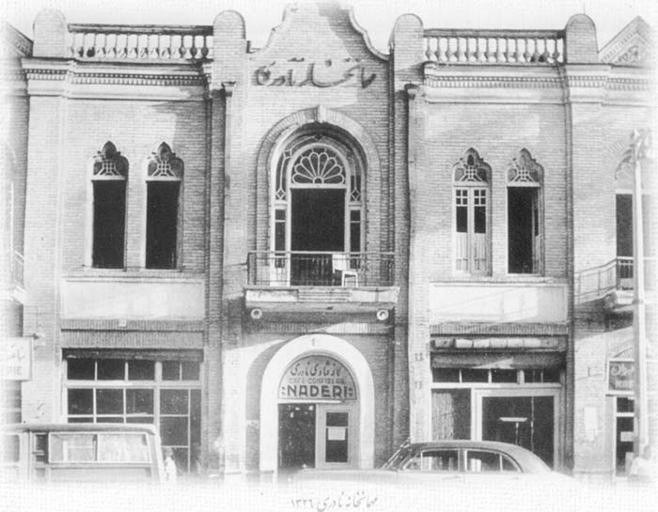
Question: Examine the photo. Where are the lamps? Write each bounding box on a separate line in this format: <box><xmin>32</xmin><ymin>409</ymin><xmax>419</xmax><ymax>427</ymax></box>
<box><xmin>330</xmin><ymin>254</ymin><xmax>359</xmax><ymax>288</ymax></box>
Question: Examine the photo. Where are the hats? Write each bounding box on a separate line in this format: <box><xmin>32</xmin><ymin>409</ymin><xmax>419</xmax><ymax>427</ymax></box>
<box><xmin>290</xmin><ymin>427</ymin><xmax>582</xmax><ymax>490</ymax></box>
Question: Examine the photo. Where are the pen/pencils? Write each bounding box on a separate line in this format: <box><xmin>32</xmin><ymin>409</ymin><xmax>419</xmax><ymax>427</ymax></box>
<box><xmin>1</xmin><ymin>417</ymin><xmax>167</xmax><ymax>487</ymax></box>
